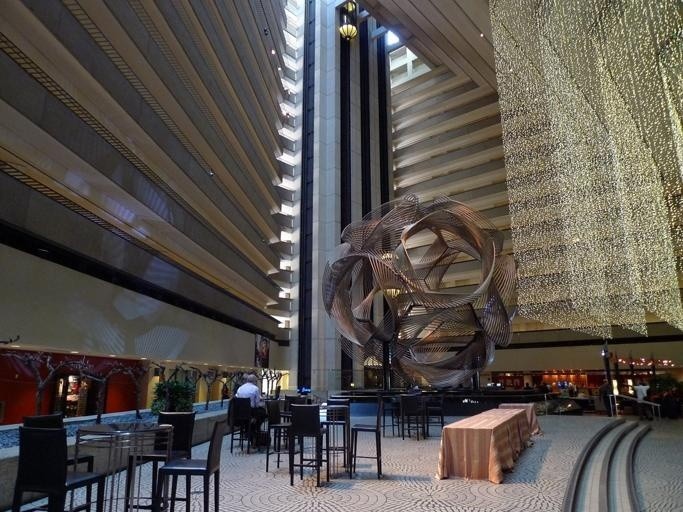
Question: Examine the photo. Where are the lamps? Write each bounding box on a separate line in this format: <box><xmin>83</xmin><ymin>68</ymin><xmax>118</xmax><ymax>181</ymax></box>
<box><xmin>338</xmin><ymin>0</ymin><xmax>359</xmax><ymax>40</ymax></box>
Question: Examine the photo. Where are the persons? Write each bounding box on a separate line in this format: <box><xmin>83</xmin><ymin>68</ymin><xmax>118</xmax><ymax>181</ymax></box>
<box><xmin>221</xmin><ymin>383</ymin><xmax>229</xmax><ymax>399</ymax></box>
<box><xmin>597</xmin><ymin>377</ymin><xmax>615</xmax><ymax>418</ymax></box>
<box><xmin>234</xmin><ymin>373</ymin><xmax>265</xmax><ymax>433</ymax></box>
<box><xmin>230</xmin><ymin>372</ymin><xmax>247</xmax><ymax>396</ymax></box>
<box><xmin>628</xmin><ymin>379</ymin><xmax>654</xmax><ymax>422</ymax></box>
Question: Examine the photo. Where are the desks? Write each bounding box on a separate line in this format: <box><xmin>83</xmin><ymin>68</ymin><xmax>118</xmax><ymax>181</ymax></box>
<box><xmin>443</xmin><ymin>408</ymin><xmax>526</xmax><ymax>484</ymax></box>
<box><xmin>70</xmin><ymin>422</ymin><xmax>175</xmax><ymax>512</ymax></box>
<box><xmin>499</xmin><ymin>402</ymin><xmax>537</xmax><ymax>436</ymax></box>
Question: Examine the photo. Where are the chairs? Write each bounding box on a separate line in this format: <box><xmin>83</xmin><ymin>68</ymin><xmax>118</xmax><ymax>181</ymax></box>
<box><xmin>24</xmin><ymin>414</ymin><xmax>95</xmax><ymax>512</ymax></box>
<box><xmin>156</xmin><ymin>419</ymin><xmax>225</xmax><ymax>512</ymax></box>
<box><xmin>13</xmin><ymin>426</ymin><xmax>105</xmax><ymax>512</ymax></box>
<box><xmin>125</xmin><ymin>411</ymin><xmax>196</xmax><ymax>511</ymax></box>
<box><xmin>226</xmin><ymin>386</ymin><xmax>444</xmax><ymax>489</ymax></box>
